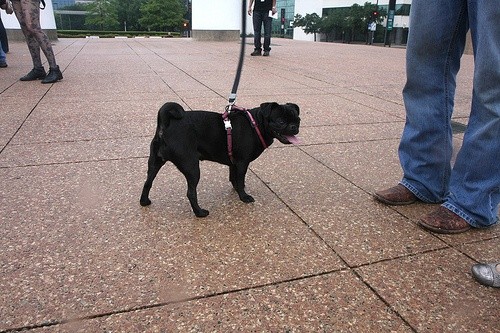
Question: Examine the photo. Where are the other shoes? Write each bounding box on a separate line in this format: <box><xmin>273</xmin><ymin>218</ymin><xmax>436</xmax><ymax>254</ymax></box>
<box><xmin>0</xmin><ymin>60</ymin><xmax>8</xmax><ymax>68</ymax></box>
<box><xmin>263</xmin><ymin>50</ymin><xmax>269</xmax><ymax>56</ymax></box>
<box><xmin>372</xmin><ymin>183</ymin><xmax>419</xmax><ymax>206</ymax></box>
<box><xmin>250</xmin><ymin>48</ymin><xmax>263</xmax><ymax>57</ymax></box>
<box><xmin>419</xmin><ymin>206</ymin><xmax>473</xmax><ymax>234</ymax></box>
<box><xmin>20</xmin><ymin>68</ymin><xmax>46</xmax><ymax>81</ymax></box>
<box><xmin>471</xmin><ymin>262</ymin><xmax>500</xmax><ymax>290</ymax></box>
<box><xmin>41</xmin><ymin>65</ymin><xmax>64</xmax><ymax>84</ymax></box>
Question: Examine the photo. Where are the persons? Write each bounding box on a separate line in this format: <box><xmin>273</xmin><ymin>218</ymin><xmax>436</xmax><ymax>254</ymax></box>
<box><xmin>470</xmin><ymin>263</ymin><xmax>500</xmax><ymax>290</ymax></box>
<box><xmin>0</xmin><ymin>0</ymin><xmax>10</xmax><ymax>67</ymax></box>
<box><xmin>373</xmin><ymin>0</ymin><xmax>500</xmax><ymax>234</ymax></box>
<box><xmin>247</xmin><ymin>0</ymin><xmax>278</xmax><ymax>56</ymax></box>
<box><xmin>11</xmin><ymin>0</ymin><xmax>63</xmax><ymax>84</ymax></box>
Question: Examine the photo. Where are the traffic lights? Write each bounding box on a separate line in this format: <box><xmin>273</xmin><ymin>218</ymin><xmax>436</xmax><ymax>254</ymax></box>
<box><xmin>372</xmin><ymin>9</ymin><xmax>378</xmax><ymax>18</ymax></box>
<box><xmin>183</xmin><ymin>22</ymin><xmax>189</xmax><ymax>31</ymax></box>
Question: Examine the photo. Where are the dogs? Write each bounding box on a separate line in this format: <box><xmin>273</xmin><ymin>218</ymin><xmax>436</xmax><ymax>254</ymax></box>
<box><xmin>140</xmin><ymin>101</ymin><xmax>301</xmax><ymax>218</ymax></box>
